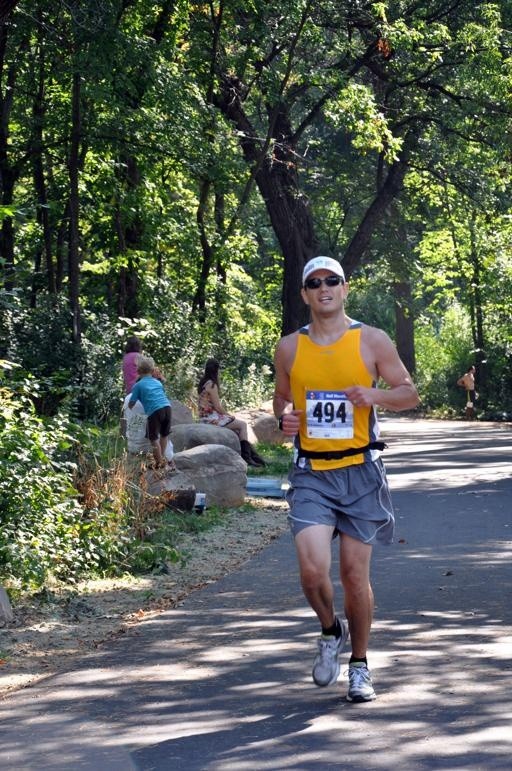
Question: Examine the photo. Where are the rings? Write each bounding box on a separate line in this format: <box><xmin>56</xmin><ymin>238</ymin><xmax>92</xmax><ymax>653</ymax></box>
<box><xmin>353</xmin><ymin>400</ymin><xmax>358</xmax><ymax>404</ymax></box>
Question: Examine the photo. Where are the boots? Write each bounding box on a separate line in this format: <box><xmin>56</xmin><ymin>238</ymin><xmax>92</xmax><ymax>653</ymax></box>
<box><xmin>240</xmin><ymin>440</ymin><xmax>273</xmax><ymax>467</ymax></box>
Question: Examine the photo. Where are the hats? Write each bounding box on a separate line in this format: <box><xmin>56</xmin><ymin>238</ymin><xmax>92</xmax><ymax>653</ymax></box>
<box><xmin>302</xmin><ymin>256</ymin><xmax>345</xmax><ymax>287</ymax></box>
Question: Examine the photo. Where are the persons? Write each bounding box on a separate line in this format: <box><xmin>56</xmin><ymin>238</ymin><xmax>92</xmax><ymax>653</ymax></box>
<box><xmin>310</xmin><ymin>391</ymin><xmax>317</xmax><ymax>400</ymax></box>
<box><xmin>128</xmin><ymin>355</ymin><xmax>171</xmax><ymax>470</ymax></box>
<box><xmin>272</xmin><ymin>253</ymin><xmax>421</xmax><ymax>706</ymax></box>
<box><xmin>119</xmin><ymin>369</ymin><xmax>174</xmax><ymax>462</ymax></box>
<box><xmin>121</xmin><ymin>335</ymin><xmax>144</xmax><ymax>395</ymax></box>
<box><xmin>196</xmin><ymin>357</ymin><xmax>267</xmax><ymax>467</ymax></box>
<box><xmin>457</xmin><ymin>365</ymin><xmax>476</xmax><ymax>420</ymax></box>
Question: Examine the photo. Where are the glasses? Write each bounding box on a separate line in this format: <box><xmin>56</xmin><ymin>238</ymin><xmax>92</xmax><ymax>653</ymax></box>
<box><xmin>306</xmin><ymin>275</ymin><xmax>341</xmax><ymax>289</ymax></box>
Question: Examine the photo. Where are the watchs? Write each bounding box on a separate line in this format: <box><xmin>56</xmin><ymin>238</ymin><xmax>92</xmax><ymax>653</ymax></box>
<box><xmin>276</xmin><ymin>413</ymin><xmax>288</xmax><ymax>432</ymax></box>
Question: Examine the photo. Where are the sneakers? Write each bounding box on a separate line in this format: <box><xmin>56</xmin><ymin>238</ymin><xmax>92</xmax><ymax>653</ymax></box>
<box><xmin>312</xmin><ymin>619</ymin><xmax>349</xmax><ymax>687</ymax></box>
<box><xmin>148</xmin><ymin>462</ymin><xmax>165</xmax><ymax>469</ymax></box>
<box><xmin>346</xmin><ymin>662</ymin><xmax>376</xmax><ymax>702</ymax></box>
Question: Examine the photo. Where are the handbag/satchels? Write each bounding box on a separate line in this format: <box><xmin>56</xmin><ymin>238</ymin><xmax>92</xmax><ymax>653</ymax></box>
<box><xmin>169</xmin><ymin>490</ymin><xmax>206</xmax><ymax>515</ymax></box>
